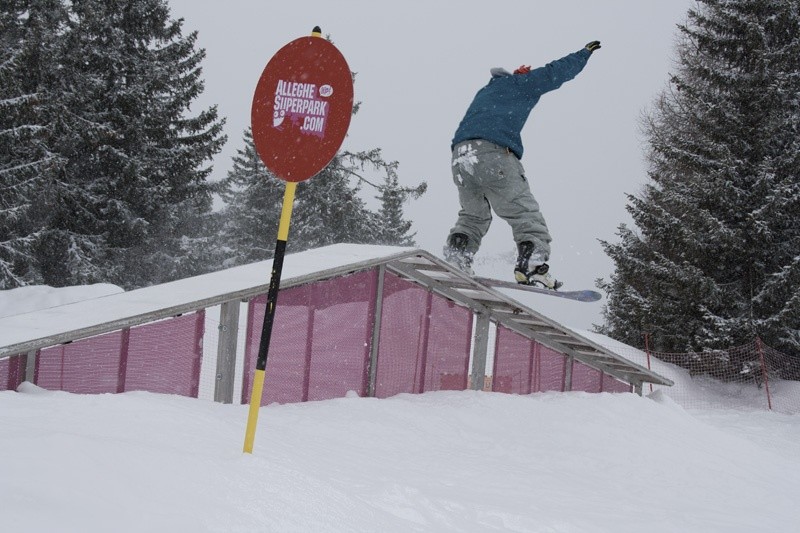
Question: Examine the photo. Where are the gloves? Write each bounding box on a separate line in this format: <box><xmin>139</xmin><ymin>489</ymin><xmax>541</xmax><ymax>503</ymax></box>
<box><xmin>586</xmin><ymin>41</ymin><xmax>601</xmax><ymax>52</ymax></box>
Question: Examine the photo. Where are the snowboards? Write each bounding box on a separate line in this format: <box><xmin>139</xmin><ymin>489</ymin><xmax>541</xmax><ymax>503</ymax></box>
<box><xmin>470</xmin><ymin>275</ymin><xmax>602</xmax><ymax>304</ymax></box>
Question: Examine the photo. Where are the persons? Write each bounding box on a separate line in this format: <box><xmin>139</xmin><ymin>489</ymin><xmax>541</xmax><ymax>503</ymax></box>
<box><xmin>443</xmin><ymin>37</ymin><xmax>601</xmax><ymax>289</ymax></box>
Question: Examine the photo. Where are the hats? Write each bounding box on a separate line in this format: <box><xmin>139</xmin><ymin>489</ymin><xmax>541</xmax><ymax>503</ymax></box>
<box><xmin>513</xmin><ymin>64</ymin><xmax>531</xmax><ymax>75</ymax></box>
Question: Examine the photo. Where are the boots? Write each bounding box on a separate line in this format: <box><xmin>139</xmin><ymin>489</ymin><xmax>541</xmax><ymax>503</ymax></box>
<box><xmin>445</xmin><ymin>232</ymin><xmax>476</xmax><ymax>276</ymax></box>
<box><xmin>514</xmin><ymin>240</ymin><xmax>564</xmax><ymax>289</ymax></box>
